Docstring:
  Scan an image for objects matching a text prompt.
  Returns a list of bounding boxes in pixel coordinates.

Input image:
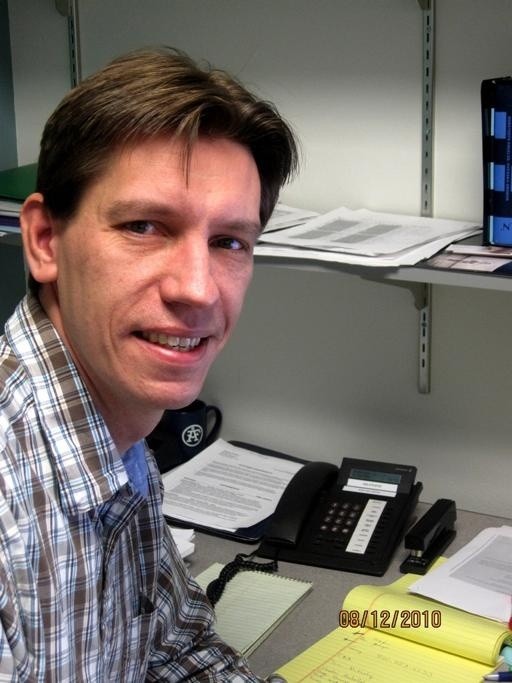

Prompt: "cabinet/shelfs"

[0,1,511,394]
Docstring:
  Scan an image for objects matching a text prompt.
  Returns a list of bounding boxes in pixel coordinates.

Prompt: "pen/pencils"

[482,671,512,683]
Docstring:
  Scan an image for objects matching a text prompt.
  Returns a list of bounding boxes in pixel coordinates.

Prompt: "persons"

[1,47,298,682]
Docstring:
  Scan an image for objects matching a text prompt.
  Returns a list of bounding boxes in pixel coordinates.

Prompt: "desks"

[162,438,512,683]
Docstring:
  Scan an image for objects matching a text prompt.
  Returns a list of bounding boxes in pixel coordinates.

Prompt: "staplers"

[399,498,457,575]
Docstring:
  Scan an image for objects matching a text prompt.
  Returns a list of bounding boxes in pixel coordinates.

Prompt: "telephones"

[205,456,424,607]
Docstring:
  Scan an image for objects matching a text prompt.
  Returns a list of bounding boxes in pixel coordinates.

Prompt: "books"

[485,95,512,246]
[2,163,40,219]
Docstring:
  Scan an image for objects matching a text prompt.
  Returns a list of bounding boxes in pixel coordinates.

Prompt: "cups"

[172,400,222,461]
